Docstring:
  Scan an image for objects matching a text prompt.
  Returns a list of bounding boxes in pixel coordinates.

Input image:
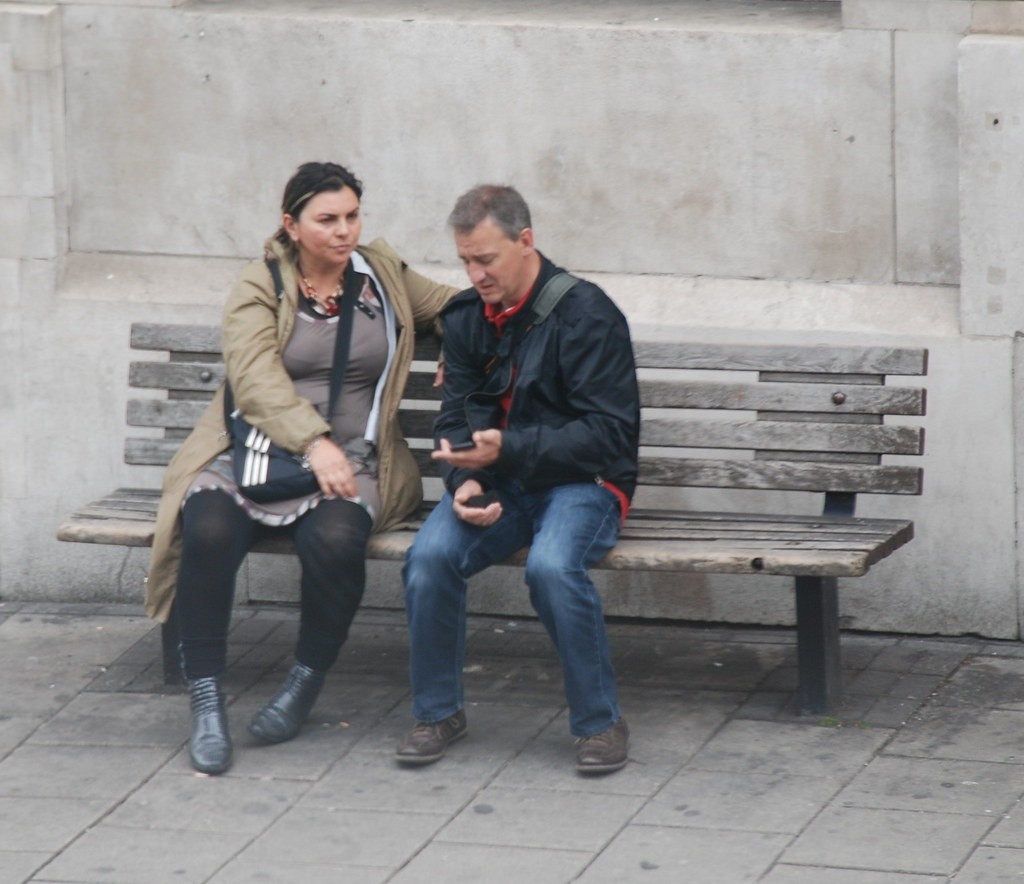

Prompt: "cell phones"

[449,442,474,452]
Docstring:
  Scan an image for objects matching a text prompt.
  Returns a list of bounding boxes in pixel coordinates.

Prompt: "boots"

[247,663,326,743]
[187,674,234,772]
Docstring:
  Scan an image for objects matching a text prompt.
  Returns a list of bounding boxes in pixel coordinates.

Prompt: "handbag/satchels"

[228,403,320,504]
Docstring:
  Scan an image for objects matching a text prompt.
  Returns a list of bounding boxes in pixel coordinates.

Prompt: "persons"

[395,187,641,773]
[144,162,464,774]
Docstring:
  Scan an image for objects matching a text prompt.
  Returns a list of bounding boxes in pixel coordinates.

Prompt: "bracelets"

[301,436,323,468]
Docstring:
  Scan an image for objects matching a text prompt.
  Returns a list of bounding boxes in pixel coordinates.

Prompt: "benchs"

[62,319,928,707]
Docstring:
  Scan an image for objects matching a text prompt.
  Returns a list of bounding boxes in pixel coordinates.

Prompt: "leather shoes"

[574,717,630,771]
[395,709,469,761]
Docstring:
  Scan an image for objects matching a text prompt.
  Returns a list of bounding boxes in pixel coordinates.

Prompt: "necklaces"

[296,262,346,309]
